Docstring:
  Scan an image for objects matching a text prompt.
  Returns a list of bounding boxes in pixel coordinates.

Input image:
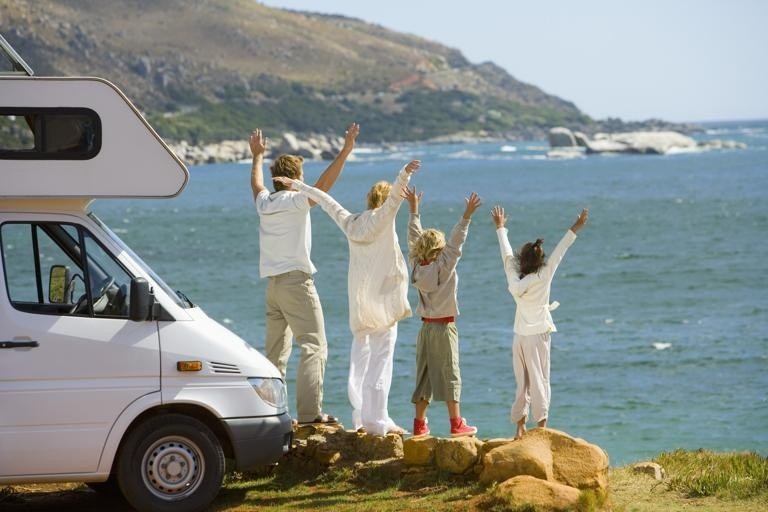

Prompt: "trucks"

[0,33,295,509]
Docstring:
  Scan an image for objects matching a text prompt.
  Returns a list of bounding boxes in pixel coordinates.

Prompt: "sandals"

[298,414,339,425]
[387,424,411,436]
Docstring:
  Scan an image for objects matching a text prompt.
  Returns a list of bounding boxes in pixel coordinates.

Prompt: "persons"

[398,183,484,437]
[269,158,421,436]
[246,121,360,424]
[489,205,590,440]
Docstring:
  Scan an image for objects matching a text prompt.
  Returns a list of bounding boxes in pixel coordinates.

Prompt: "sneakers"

[413,417,431,437]
[449,416,477,437]
